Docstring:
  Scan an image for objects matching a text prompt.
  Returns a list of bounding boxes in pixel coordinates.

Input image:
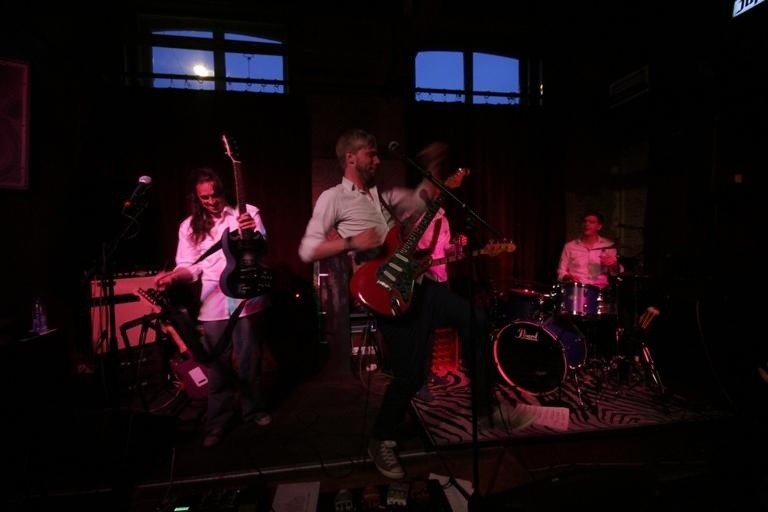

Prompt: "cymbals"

[589,241,637,251]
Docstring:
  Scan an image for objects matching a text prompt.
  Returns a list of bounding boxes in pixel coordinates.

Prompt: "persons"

[148,164,274,449]
[298,127,492,477]
[552,207,621,394]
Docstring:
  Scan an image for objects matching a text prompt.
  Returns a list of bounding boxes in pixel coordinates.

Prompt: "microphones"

[122,174,153,208]
[614,222,637,232]
[389,139,432,178]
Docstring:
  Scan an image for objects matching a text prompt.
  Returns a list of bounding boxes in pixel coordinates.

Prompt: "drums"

[558,282,598,322]
[495,288,557,326]
[597,288,618,317]
[493,318,587,396]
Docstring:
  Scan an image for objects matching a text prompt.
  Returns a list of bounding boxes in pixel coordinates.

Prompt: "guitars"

[220,134,274,298]
[350,169,471,319]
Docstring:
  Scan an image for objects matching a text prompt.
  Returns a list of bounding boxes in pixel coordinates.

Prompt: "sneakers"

[203,430,218,447]
[254,413,271,426]
[367,441,405,480]
